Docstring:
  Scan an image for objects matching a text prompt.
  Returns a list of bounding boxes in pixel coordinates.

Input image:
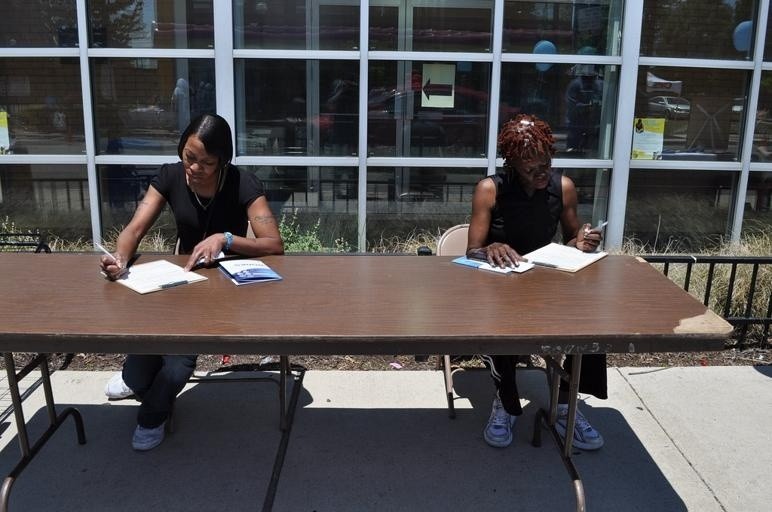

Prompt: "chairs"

[165,221,291,431]
[436,224,553,418]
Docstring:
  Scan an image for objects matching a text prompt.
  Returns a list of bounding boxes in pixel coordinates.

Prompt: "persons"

[467,114,607,450]
[566,72,605,149]
[99,113,284,451]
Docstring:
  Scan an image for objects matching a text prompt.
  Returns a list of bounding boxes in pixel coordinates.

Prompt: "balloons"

[532,38,558,73]
[732,20,753,51]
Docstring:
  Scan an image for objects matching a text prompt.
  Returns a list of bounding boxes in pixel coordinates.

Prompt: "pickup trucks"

[2,101,303,216]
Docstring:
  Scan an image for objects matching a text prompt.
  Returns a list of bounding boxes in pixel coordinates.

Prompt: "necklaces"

[189,168,222,210]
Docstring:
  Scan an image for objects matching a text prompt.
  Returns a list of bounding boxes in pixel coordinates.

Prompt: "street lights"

[530,37,560,97]
[730,19,754,106]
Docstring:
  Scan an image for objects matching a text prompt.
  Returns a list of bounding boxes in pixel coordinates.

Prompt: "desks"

[0,250,733,512]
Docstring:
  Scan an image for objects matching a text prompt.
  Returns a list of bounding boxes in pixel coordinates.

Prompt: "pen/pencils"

[584,221,609,235]
[95,242,130,274]
[532,262,556,268]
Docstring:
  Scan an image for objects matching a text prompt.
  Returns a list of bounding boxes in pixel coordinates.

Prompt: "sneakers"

[483,391,519,451]
[129,420,169,452]
[546,402,607,455]
[103,371,136,400]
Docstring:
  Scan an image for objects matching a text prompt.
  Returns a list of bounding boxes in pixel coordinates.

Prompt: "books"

[450,251,537,275]
[214,257,282,286]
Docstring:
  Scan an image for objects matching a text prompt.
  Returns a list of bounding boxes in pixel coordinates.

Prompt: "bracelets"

[224,232,233,252]
